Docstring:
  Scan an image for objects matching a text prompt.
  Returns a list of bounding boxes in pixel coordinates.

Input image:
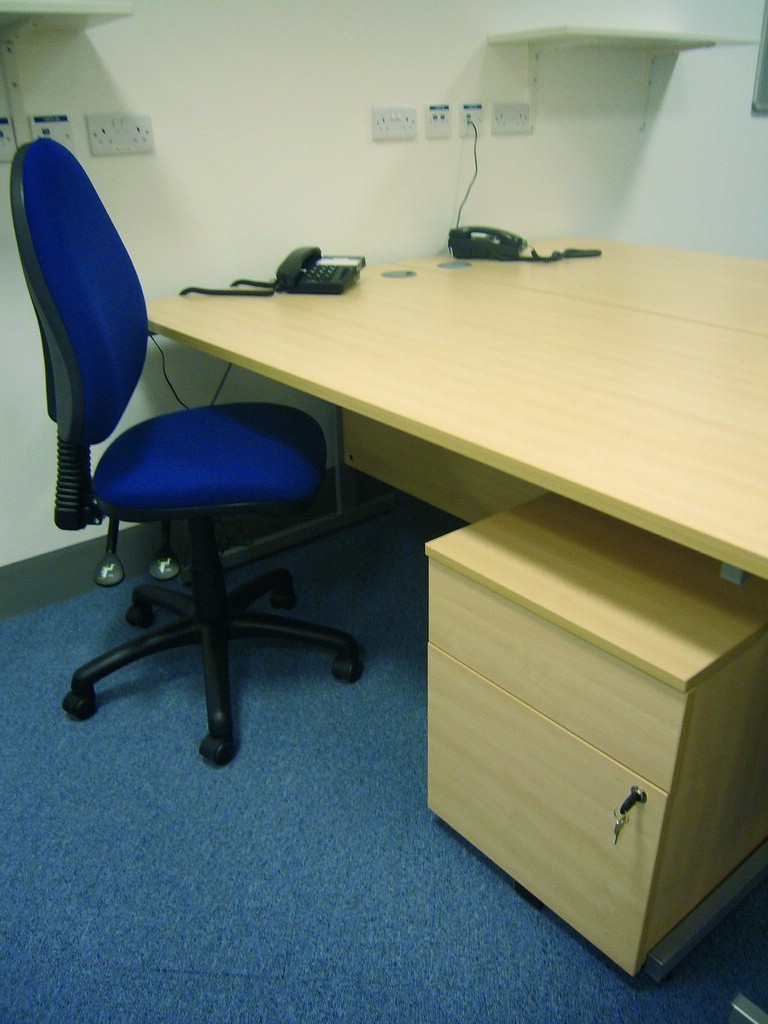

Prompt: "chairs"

[10,138,367,768]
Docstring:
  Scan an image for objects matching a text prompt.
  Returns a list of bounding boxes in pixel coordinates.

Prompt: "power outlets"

[458,102,483,138]
[85,113,155,158]
[489,102,530,135]
[371,106,417,141]
[35,114,73,149]
[426,103,451,138]
[0,116,15,162]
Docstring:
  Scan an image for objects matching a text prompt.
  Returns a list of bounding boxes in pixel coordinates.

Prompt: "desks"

[142,247,768,581]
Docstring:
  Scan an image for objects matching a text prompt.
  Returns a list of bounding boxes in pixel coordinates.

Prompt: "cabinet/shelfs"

[422,493,768,978]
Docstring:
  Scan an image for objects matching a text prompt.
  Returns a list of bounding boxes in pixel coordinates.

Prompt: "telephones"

[275,246,368,298]
[448,224,523,262]
